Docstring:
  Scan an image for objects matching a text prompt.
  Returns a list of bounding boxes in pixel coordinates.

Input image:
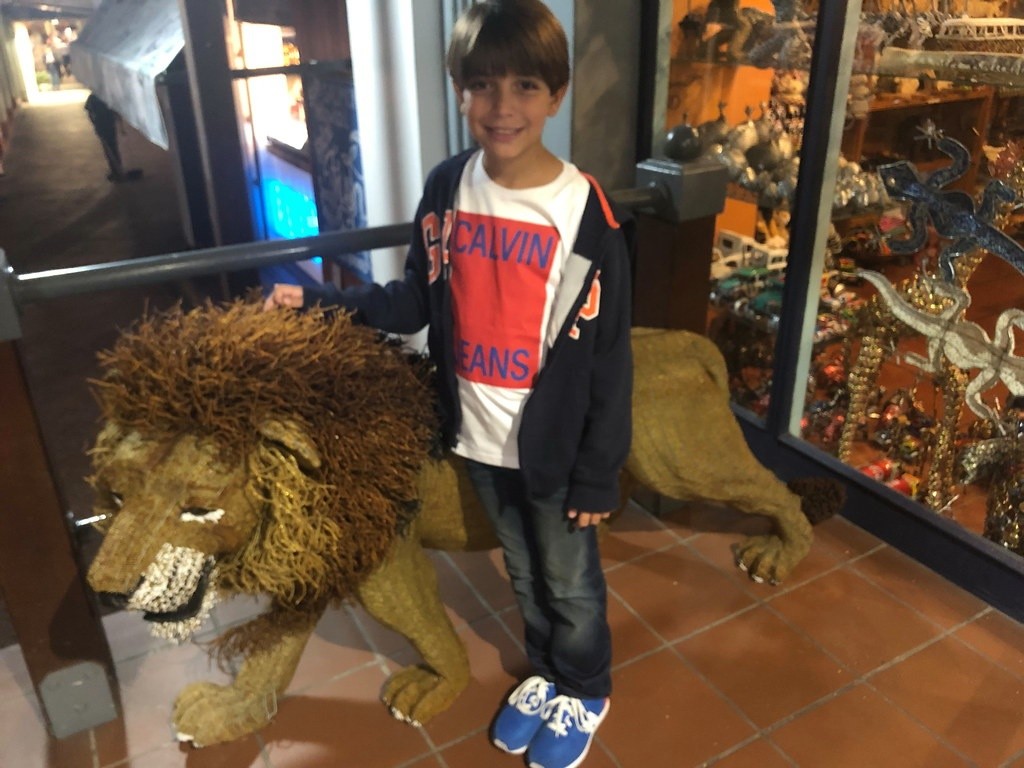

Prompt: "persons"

[42,24,78,91]
[85,93,125,174]
[261,1,634,768]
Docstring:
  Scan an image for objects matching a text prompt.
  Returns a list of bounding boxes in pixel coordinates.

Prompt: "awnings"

[69,0,186,151]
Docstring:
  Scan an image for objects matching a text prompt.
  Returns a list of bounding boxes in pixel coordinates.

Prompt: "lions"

[87,288,845,750]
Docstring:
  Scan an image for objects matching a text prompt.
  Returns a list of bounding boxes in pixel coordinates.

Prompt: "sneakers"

[494,673,560,755]
[527,691,610,768]
